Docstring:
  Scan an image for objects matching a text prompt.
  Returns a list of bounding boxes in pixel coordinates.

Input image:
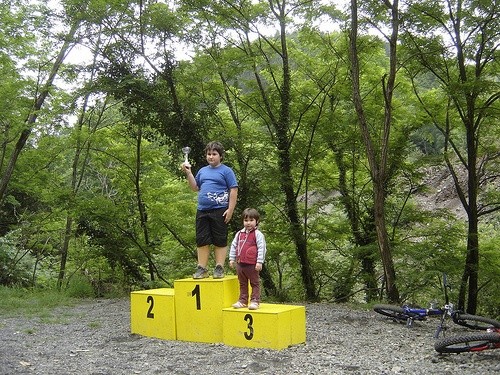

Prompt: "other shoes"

[193,265,209,278]
[213,263,224,278]
[248,302,260,310]
[232,302,248,309]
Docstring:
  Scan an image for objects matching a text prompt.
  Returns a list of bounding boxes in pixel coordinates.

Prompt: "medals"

[236,256,240,264]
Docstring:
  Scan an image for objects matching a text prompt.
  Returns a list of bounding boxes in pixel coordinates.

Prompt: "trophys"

[181,147,192,169]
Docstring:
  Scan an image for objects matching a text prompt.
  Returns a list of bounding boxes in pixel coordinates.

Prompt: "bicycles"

[434,334,500,354]
[372,273,500,338]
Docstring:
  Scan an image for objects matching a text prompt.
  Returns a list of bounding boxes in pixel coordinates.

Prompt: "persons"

[228,208,267,310]
[181,141,239,279]
